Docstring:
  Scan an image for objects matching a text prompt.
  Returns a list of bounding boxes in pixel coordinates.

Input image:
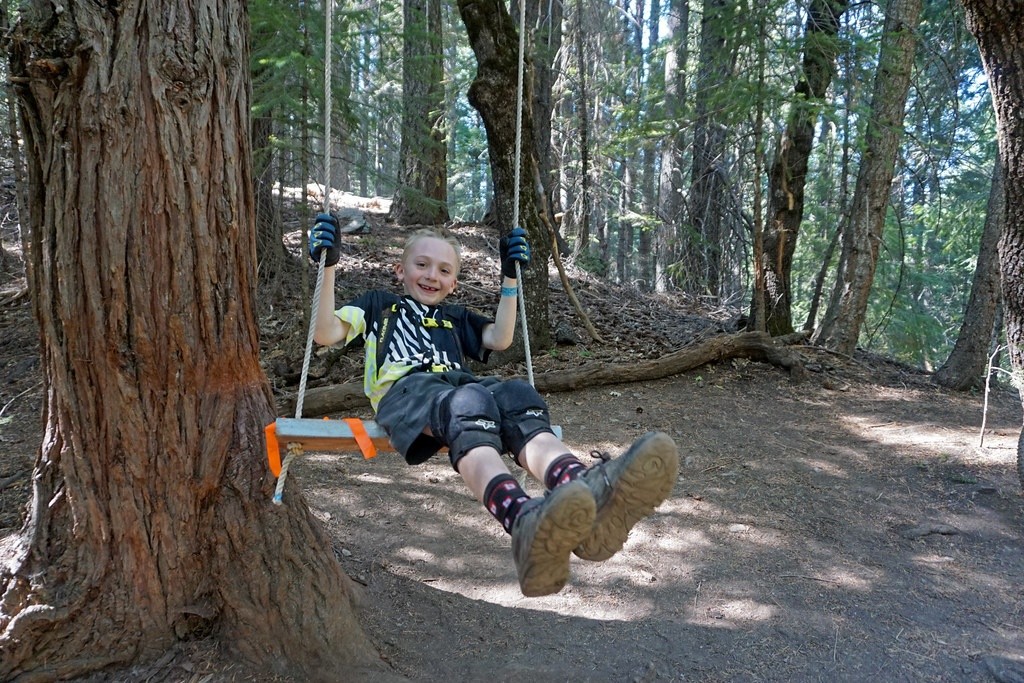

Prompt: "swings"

[264,1,565,456]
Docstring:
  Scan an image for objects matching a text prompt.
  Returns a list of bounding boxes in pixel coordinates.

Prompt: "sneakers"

[512,480,597,597]
[572,430,678,562]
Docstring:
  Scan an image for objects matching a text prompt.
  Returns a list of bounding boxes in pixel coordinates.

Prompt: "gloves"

[309,210,341,267]
[499,227,531,279]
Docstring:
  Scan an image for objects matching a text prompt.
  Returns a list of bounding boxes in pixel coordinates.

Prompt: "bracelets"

[501,283,518,297]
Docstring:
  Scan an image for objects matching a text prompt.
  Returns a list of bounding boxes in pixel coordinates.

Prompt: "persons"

[310,212,678,597]
